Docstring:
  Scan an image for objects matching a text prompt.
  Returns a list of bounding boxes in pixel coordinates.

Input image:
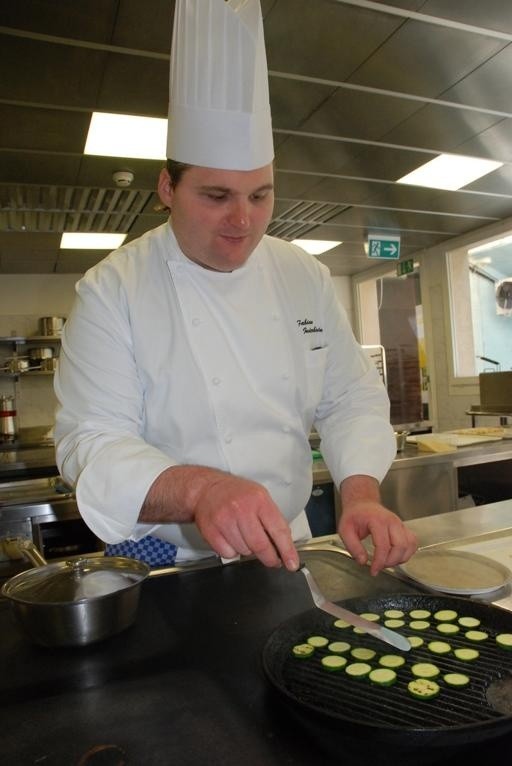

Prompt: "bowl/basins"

[37,316,66,336]
[394,428,410,453]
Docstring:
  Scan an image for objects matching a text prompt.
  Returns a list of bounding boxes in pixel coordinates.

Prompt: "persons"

[52,154,418,580]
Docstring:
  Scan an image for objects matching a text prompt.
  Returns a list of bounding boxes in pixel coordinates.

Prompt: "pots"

[18,356,59,371]
[260,591,512,745]
[4,346,54,361]
[1,537,154,653]
[0,352,31,374]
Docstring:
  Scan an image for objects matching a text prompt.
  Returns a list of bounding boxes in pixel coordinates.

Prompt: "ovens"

[28,508,108,567]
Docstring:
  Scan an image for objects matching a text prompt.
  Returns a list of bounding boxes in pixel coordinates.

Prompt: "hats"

[166,0,275,171]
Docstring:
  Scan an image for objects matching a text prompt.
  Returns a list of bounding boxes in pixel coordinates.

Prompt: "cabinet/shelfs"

[0,335,62,376]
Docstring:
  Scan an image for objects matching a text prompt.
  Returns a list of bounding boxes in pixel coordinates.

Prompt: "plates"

[396,548,511,596]
